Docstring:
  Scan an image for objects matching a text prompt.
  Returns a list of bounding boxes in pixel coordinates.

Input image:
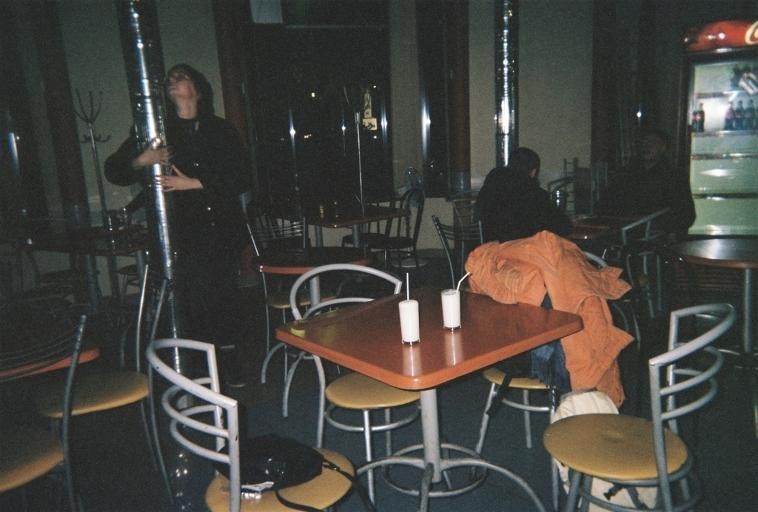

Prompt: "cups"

[396,299,421,346]
[445,333,466,367]
[440,289,462,330]
[400,347,422,379]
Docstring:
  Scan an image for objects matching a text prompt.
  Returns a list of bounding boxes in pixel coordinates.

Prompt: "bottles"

[105,204,130,232]
[722,98,757,130]
[692,104,707,133]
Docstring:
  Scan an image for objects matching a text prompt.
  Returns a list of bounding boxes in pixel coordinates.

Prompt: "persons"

[473,146,577,244]
[592,124,695,282]
[104,64,251,387]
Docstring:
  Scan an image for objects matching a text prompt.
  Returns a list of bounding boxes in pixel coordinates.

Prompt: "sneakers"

[216,345,248,389]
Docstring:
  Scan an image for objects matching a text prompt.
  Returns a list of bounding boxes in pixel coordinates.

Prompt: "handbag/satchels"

[210,430,325,496]
